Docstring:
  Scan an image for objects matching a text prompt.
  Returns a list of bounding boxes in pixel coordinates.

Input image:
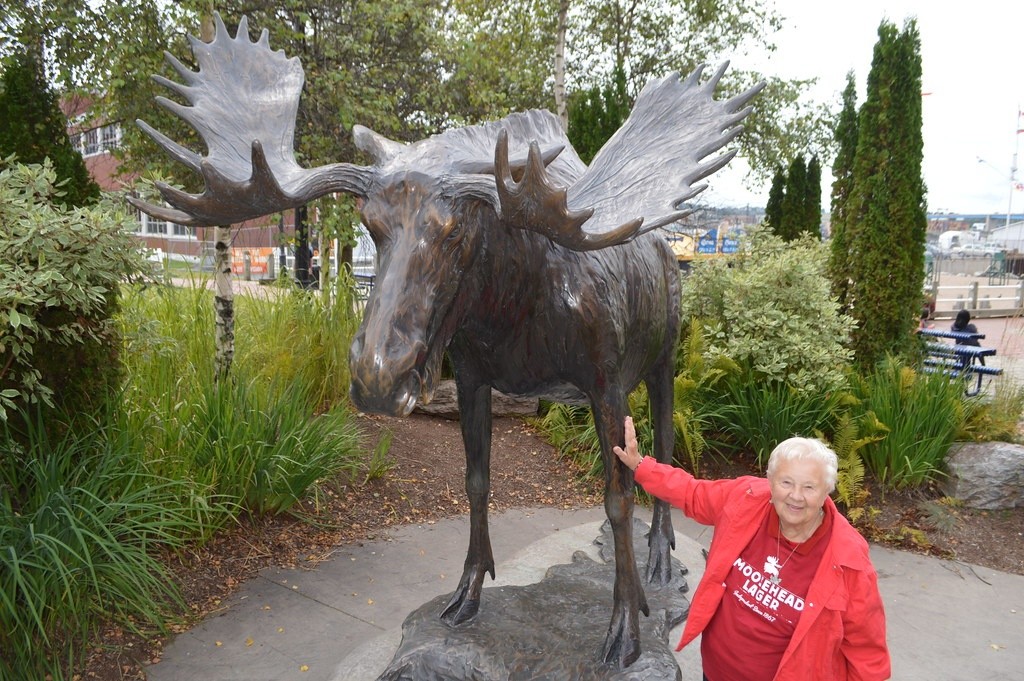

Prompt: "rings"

[635,435,637,438]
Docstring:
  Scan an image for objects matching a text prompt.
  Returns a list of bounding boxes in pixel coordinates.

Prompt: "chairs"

[330,275,374,297]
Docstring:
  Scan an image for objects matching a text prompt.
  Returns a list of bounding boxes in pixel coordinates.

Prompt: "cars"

[922,227,1024,259]
[266,254,376,281]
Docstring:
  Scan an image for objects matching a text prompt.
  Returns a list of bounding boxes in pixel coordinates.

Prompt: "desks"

[354,272,377,299]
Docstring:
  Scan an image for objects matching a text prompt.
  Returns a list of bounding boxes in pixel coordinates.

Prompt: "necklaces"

[769,506,823,588]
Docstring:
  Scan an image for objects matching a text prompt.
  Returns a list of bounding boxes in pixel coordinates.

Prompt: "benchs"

[917,328,1004,396]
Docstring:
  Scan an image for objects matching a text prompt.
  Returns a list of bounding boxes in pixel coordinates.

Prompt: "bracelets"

[634,457,644,470]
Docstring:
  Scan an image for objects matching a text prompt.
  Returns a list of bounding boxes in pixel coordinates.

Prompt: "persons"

[951,310,979,379]
[916,307,940,343]
[613,416,891,681]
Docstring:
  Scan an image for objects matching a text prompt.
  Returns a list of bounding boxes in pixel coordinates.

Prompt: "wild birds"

[123,8,770,667]
